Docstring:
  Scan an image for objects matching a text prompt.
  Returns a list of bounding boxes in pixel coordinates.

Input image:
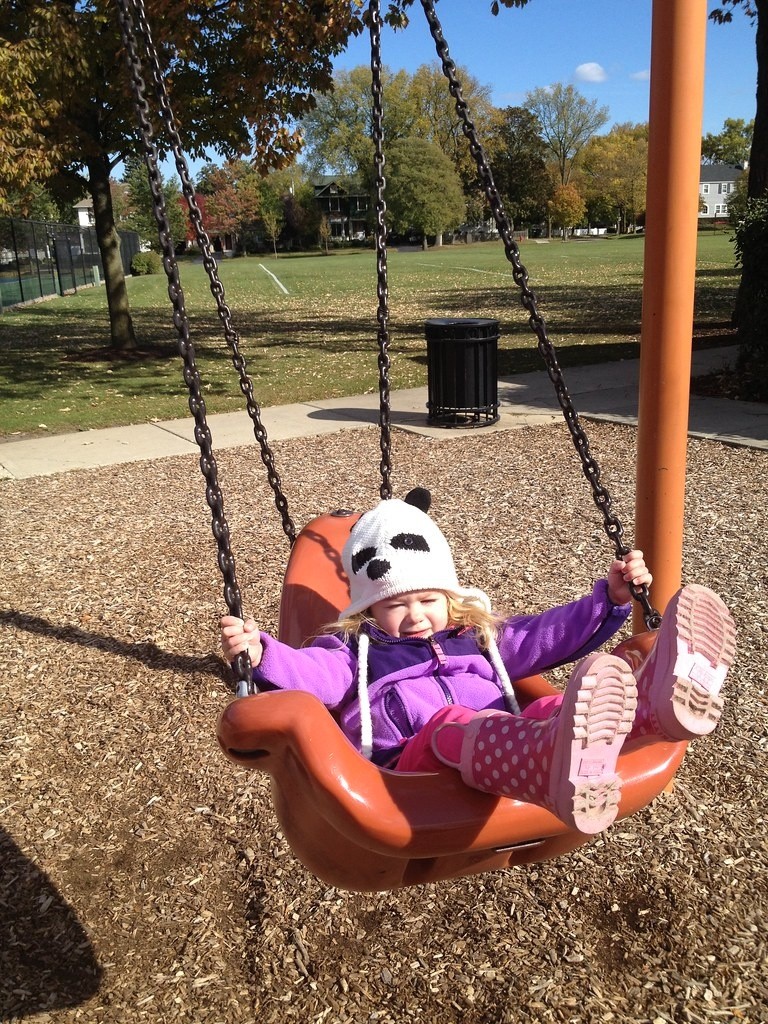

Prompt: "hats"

[338,487,491,624]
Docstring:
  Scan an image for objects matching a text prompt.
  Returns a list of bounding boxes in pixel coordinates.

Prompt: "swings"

[116,0,691,891]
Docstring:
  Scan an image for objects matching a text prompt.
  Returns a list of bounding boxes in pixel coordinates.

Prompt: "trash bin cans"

[424,317,502,429]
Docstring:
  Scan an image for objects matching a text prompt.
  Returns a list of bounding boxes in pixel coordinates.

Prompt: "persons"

[222,487,737,834]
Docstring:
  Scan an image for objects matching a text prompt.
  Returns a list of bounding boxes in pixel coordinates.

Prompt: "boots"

[548,582,737,743]
[429,652,639,835]
